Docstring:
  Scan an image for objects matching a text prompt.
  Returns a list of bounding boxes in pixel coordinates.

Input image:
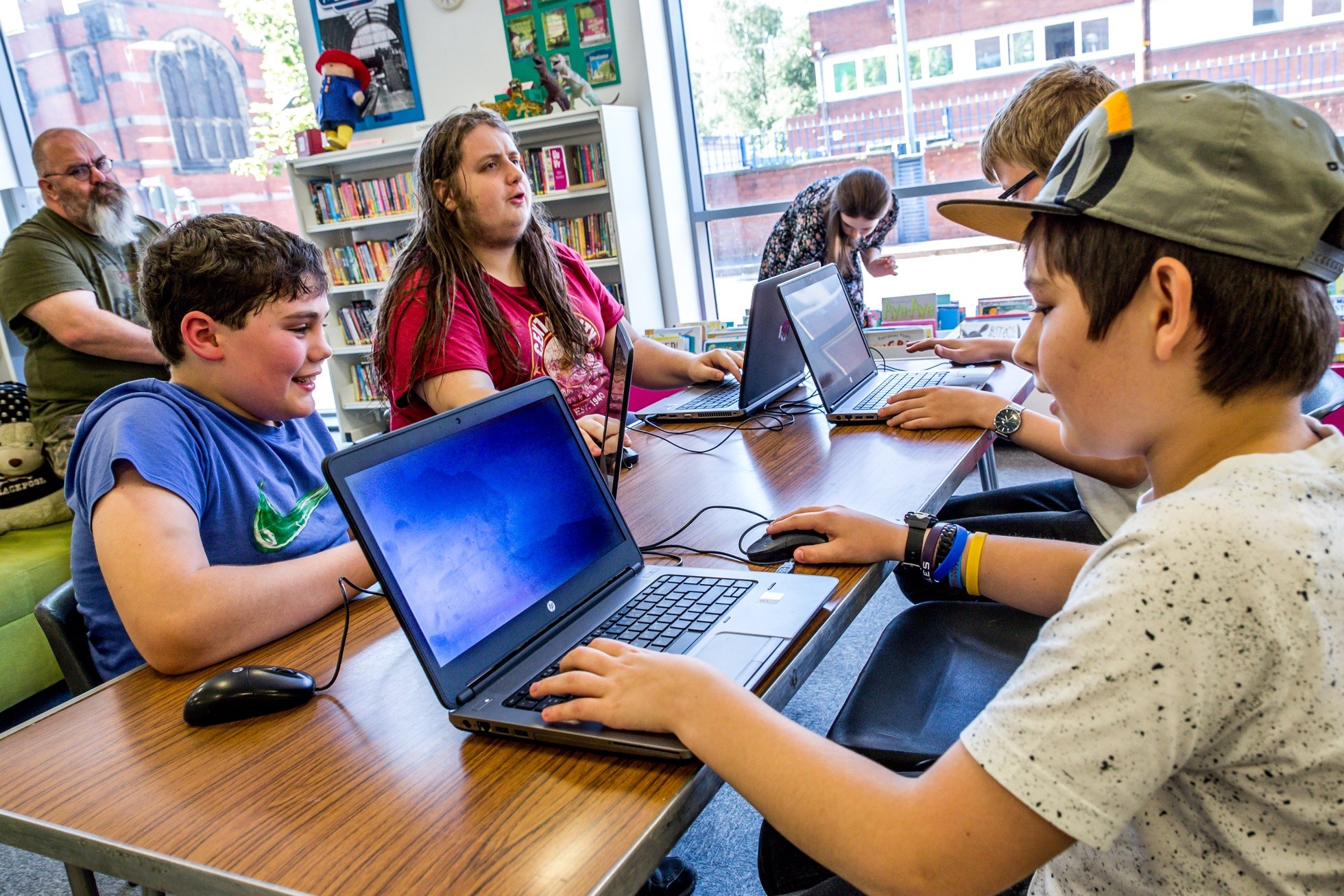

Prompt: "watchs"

[901,510,940,575]
[992,402,1027,442]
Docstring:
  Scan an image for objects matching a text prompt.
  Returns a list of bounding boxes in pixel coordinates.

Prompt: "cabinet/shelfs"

[286,102,665,444]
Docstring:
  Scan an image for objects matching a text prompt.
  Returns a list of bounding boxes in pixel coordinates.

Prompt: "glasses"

[43,157,113,181]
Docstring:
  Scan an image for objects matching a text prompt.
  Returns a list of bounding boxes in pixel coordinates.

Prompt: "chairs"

[828,601,1043,767]
[34,579,102,696]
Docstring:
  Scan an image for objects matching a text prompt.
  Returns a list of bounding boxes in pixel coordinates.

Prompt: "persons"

[0,125,170,476]
[877,61,1154,543]
[67,215,376,676]
[528,79,1344,895]
[757,167,900,328]
[371,107,745,457]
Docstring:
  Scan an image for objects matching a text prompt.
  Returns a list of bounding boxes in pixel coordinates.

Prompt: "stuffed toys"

[0,380,74,536]
[315,49,371,150]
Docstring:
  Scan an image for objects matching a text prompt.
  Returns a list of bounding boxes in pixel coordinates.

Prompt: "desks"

[0,361,1034,896]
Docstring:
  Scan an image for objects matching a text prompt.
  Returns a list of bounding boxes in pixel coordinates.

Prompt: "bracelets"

[921,522,987,597]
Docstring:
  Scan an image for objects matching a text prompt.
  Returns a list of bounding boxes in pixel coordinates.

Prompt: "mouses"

[950,352,1002,365]
[182,664,315,727]
[746,529,829,565]
[591,440,639,470]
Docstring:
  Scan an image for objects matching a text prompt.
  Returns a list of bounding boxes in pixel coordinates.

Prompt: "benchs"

[0,521,78,713]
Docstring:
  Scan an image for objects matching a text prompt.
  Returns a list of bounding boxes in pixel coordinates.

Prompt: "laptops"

[633,261,822,424]
[776,262,995,424]
[319,375,840,761]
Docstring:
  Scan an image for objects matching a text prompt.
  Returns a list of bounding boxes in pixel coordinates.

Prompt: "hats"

[936,79,1344,283]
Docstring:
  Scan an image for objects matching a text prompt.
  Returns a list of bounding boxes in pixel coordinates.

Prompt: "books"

[641,313,749,356]
[862,290,1036,349]
[307,143,623,403]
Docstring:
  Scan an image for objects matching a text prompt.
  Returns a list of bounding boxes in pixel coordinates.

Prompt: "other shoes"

[635,856,697,896]
